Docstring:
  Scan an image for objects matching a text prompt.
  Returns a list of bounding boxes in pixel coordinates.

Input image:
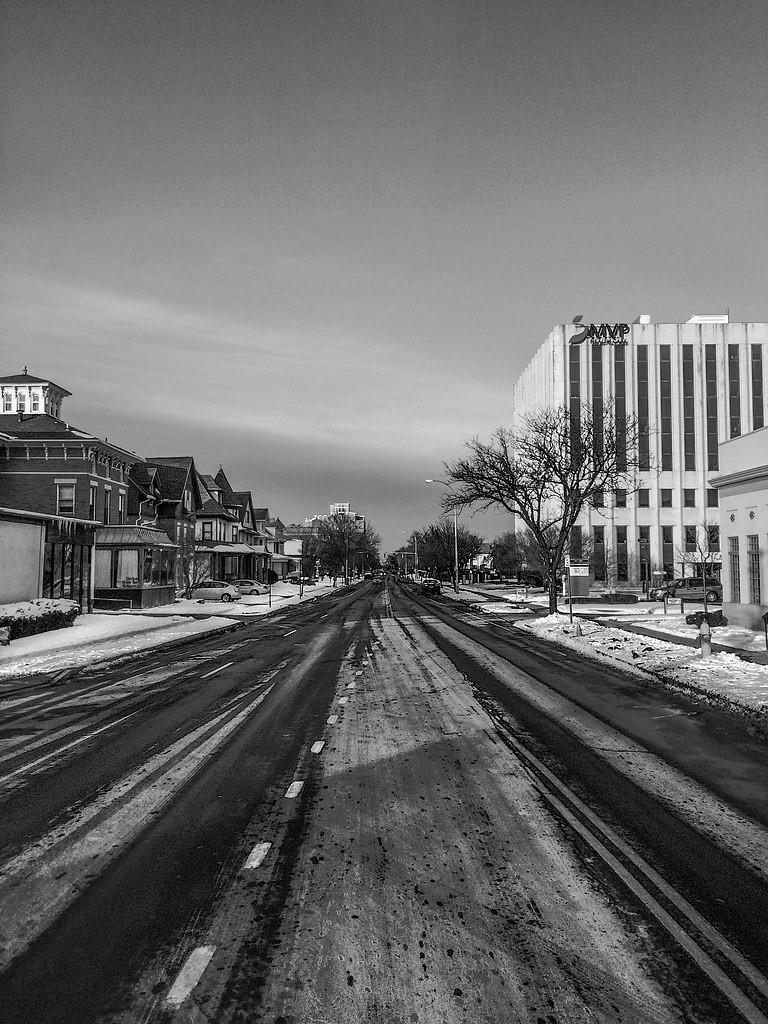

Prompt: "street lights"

[424,479,460,593]
[401,532,417,577]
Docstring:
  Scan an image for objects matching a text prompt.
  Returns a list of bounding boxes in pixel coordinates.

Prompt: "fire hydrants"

[697,618,712,657]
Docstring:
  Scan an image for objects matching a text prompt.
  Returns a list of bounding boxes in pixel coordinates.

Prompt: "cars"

[379,572,387,577]
[372,578,382,585]
[284,576,298,584]
[420,579,441,594]
[651,576,723,602]
[364,573,372,580]
[176,579,241,602]
[229,579,270,596]
